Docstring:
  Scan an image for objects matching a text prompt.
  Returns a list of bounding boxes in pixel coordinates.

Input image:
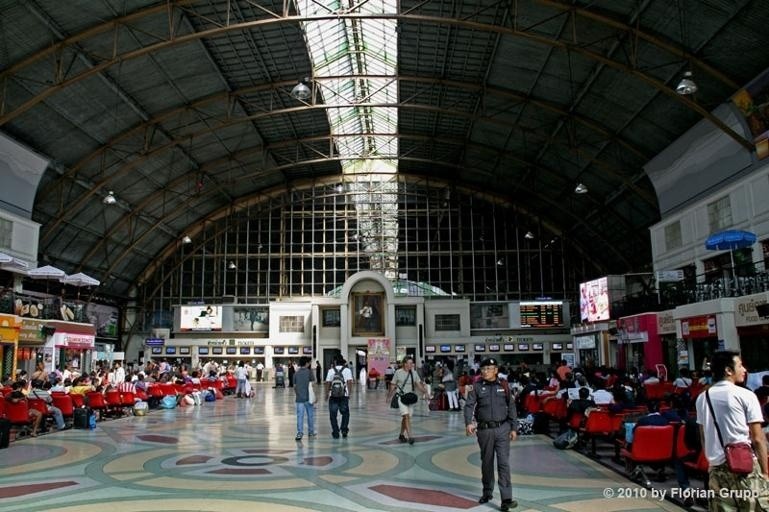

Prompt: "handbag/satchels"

[123,387,225,416]
[517,415,534,436]
[400,392,419,406]
[389,394,399,409]
[723,443,755,475]
[307,381,317,405]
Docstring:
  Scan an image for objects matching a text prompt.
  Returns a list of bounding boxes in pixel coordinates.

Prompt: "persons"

[596,284,608,319]
[580,286,590,323]
[328,350,768,482]
[293,355,318,440]
[313,360,322,386]
[695,350,769,512]
[0,354,295,438]
[359,300,373,332]
[324,354,354,439]
[182,304,269,333]
[463,359,519,512]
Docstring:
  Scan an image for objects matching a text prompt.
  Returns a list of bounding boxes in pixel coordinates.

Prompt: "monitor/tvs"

[474,344,543,352]
[166,347,176,353]
[199,347,264,354]
[180,347,190,354]
[303,347,312,354]
[274,347,284,354]
[552,342,573,350]
[152,347,162,354]
[288,347,299,354]
[454,344,464,352]
[440,345,450,352]
[425,345,436,353]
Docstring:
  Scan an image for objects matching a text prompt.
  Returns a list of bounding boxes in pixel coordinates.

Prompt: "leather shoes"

[500,497,518,512]
[479,494,495,504]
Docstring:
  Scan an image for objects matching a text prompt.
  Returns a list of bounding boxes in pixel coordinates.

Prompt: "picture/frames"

[152,346,312,354]
[425,342,573,352]
[350,291,385,336]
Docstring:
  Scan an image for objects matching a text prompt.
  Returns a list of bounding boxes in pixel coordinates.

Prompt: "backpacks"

[330,365,348,399]
[552,428,579,450]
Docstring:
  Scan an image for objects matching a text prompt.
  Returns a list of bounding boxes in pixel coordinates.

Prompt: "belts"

[481,415,511,429]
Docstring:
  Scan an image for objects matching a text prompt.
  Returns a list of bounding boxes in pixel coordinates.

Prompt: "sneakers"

[332,430,349,439]
[408,437,414,444]
[399,432,406,443]
[57,424,72,430]
[308,432,318,437]
[295,433,302,440]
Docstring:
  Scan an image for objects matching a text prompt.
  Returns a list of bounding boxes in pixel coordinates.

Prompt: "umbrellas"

[0,252,28,273]
[705,229,757,276]
[28,264,67,298]
[59,272,100,301]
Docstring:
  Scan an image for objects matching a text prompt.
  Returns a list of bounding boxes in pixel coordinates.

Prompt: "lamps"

[182,235,192,244]
[574,181,588,194]
[524,230,534,239]
[228,261,236,269]
[497,257,505,265]
[102,190,117,205]
[675,67,698,94]
[290,76,311,100]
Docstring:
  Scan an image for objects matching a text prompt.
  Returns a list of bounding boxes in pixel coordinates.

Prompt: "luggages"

[72,407,88,430]
[533,412,550,435]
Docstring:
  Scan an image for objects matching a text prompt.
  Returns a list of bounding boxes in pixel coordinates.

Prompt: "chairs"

[525,381,769,512]
[0,374,237,438]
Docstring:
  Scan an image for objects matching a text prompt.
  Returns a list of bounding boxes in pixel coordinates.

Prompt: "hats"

[479,358,497,367]
